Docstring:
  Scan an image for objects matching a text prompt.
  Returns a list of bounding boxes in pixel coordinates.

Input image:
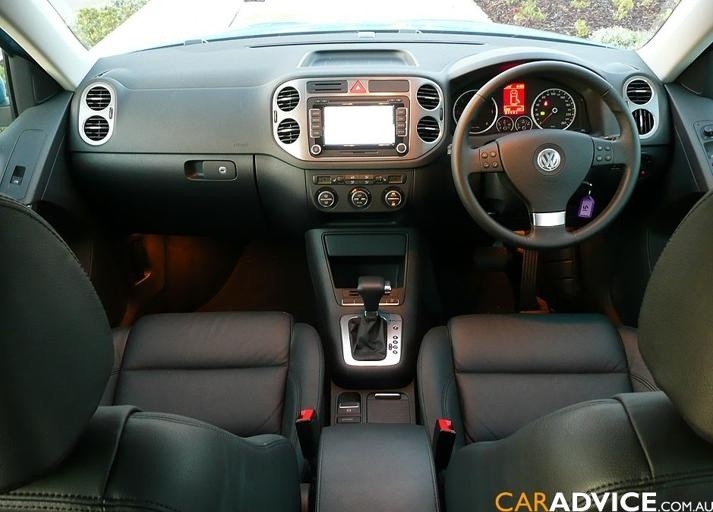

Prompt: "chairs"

[0,192,713,512]
